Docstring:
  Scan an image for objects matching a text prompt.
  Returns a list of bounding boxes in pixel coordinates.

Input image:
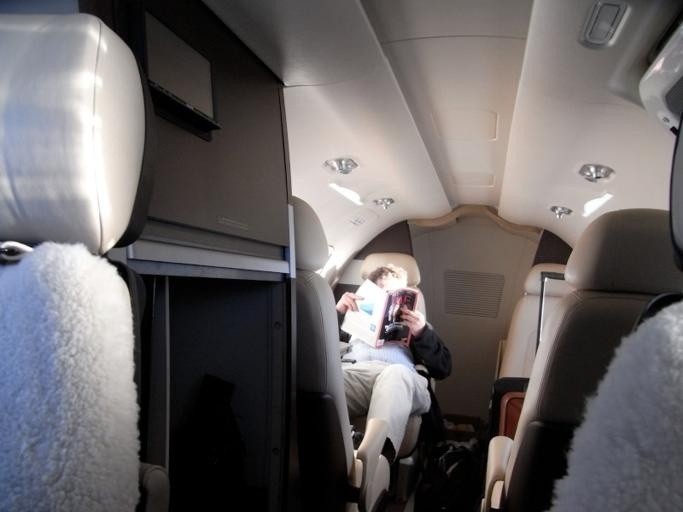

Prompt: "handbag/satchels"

[413,443,477,511]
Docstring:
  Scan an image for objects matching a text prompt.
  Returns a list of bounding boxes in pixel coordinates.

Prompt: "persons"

[336,263,452,466]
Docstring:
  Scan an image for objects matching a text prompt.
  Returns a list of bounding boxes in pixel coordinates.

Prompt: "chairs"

[0,12,146,512]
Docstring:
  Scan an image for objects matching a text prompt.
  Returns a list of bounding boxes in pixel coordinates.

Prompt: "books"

[339,278,419,350]
